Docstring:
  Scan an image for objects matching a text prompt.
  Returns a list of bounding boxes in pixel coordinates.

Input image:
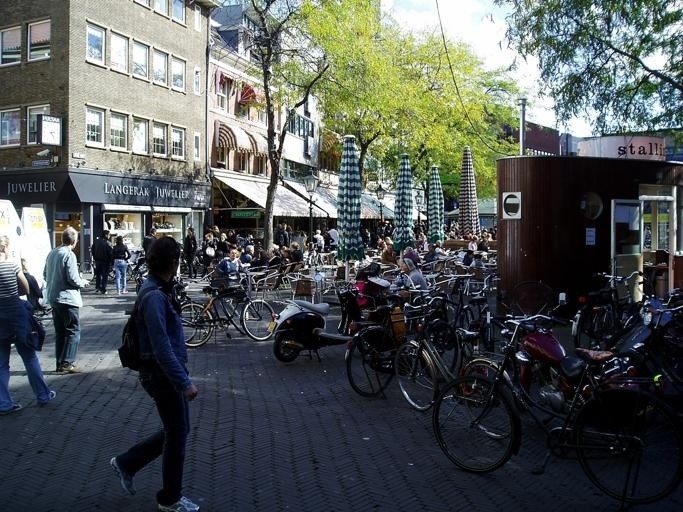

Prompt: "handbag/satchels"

[206,246,215,256]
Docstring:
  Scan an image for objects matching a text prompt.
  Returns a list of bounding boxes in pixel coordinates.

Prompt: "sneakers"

[56,363,83,372]
[159,497,200,512]
[111,458,136,497]
[50,391,56,400]
[0,405,22,415]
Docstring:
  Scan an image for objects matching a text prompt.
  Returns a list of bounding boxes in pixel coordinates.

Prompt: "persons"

[19,258,43,315]
[251,222,339,293]
[90,216,254,293]
[0,232,56,417]
[41,225,90,374]
[110,232,202,511]
[366,220,494,290]
[643,224,652,250]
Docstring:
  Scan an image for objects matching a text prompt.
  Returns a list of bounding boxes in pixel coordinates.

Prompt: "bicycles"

[430,314,683,512]
[79,247,149,296]
[172,243,683,441]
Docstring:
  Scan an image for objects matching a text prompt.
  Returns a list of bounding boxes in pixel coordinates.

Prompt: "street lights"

[414,190,424,227]
[375,184,387,241]
[302,168,320,251]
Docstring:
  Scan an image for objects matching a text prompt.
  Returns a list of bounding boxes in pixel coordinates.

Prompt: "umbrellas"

[458,144,484,240]
[337,133,365,287]
[393,153,417,264]
[426,163,445,251]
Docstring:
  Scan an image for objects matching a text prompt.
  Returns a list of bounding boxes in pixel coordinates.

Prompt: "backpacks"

[118,287,169,370]
